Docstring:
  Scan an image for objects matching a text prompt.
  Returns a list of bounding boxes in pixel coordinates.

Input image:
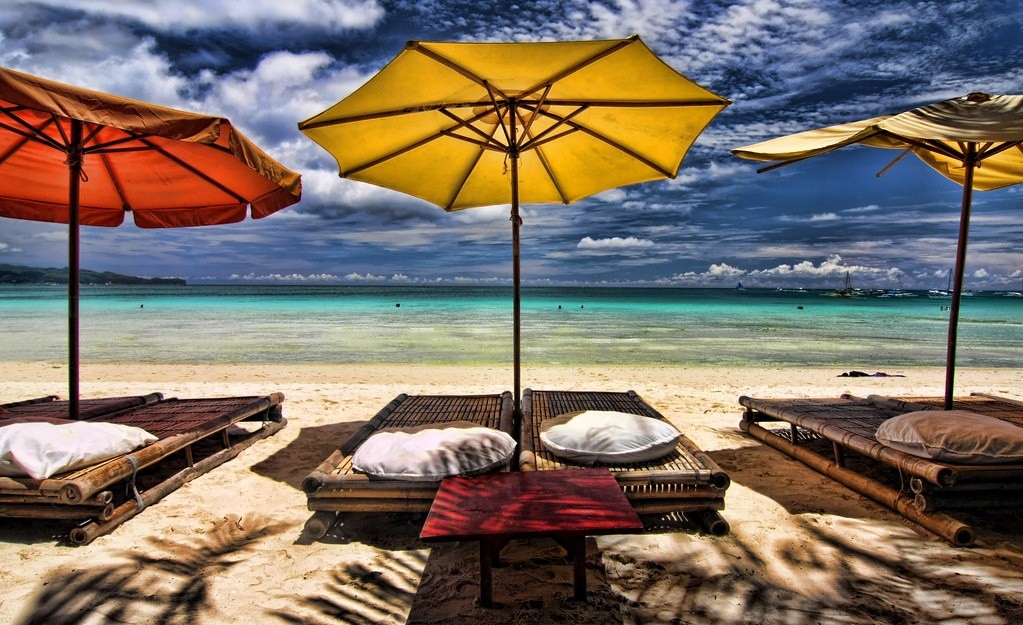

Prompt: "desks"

[420,467,643,610]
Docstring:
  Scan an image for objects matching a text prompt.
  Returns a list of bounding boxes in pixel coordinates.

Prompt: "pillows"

[350,420,517,482]
[0,417,159,482]
[540,410,685,467]
[874,409,1023,466]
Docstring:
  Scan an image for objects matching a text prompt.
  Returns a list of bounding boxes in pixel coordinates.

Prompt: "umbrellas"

[298,35,732,426]
[734,92,1023,412]
[0,66,302,421]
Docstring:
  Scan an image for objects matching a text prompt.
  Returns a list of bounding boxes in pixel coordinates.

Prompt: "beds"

[0,391,288,543]
[738,391,1023,545]
[521,386,730,535]
[301,391,513,540]
